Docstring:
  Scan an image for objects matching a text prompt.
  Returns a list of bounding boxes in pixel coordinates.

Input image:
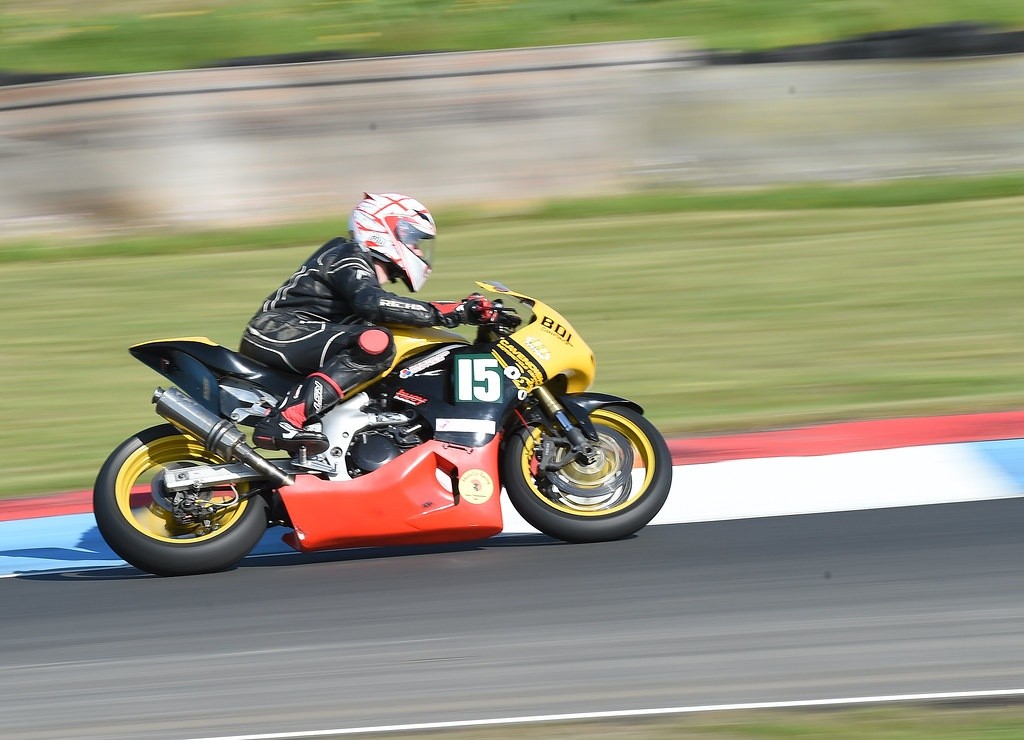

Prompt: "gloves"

[428,292,494,328]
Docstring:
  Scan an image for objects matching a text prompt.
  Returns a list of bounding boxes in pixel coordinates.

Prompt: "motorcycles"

[92,282,673,575]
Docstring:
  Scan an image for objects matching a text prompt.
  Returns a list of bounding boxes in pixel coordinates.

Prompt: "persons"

[239,190,498,455]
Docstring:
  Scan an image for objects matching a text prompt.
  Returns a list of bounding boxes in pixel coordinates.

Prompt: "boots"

[252,373,344,455]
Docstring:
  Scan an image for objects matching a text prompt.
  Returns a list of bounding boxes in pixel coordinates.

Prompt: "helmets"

[347,192,437,293]
